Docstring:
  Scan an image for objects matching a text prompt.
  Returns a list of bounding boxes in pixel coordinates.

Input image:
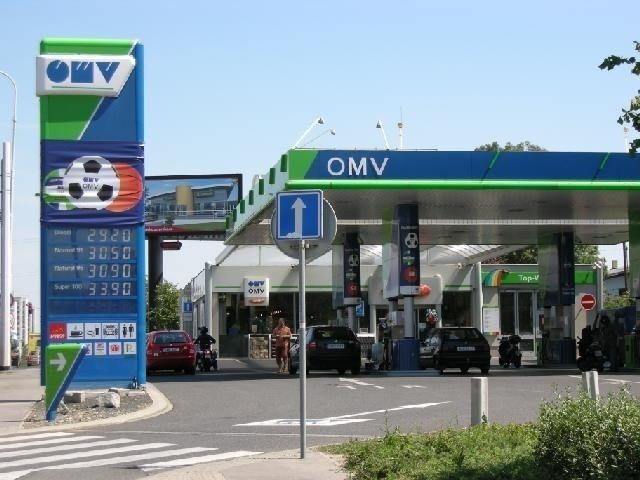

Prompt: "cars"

[421,326,494,379]
[27,350,42,366]
[145,330,197,377]
[285,323,363,375]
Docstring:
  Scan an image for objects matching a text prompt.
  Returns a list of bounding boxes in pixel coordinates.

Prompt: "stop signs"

[580,293,595,311]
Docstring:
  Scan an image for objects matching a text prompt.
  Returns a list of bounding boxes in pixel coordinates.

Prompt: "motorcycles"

[496,334,524,370]
[192,337,218,373]
[576,327,606,374]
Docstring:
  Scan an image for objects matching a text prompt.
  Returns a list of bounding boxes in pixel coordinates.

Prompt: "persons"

[195,327,214,347]
[272,318,292,371]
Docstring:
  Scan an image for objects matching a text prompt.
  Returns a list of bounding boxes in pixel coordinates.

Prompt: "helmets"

[200,326,209,334]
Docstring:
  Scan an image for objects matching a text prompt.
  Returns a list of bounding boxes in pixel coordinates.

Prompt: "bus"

[10,336,24,368]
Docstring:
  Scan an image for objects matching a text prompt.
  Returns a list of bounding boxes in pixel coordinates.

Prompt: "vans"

[593,307,628,373]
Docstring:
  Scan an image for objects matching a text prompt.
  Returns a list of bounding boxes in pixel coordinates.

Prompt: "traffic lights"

[27,302,33,316]
